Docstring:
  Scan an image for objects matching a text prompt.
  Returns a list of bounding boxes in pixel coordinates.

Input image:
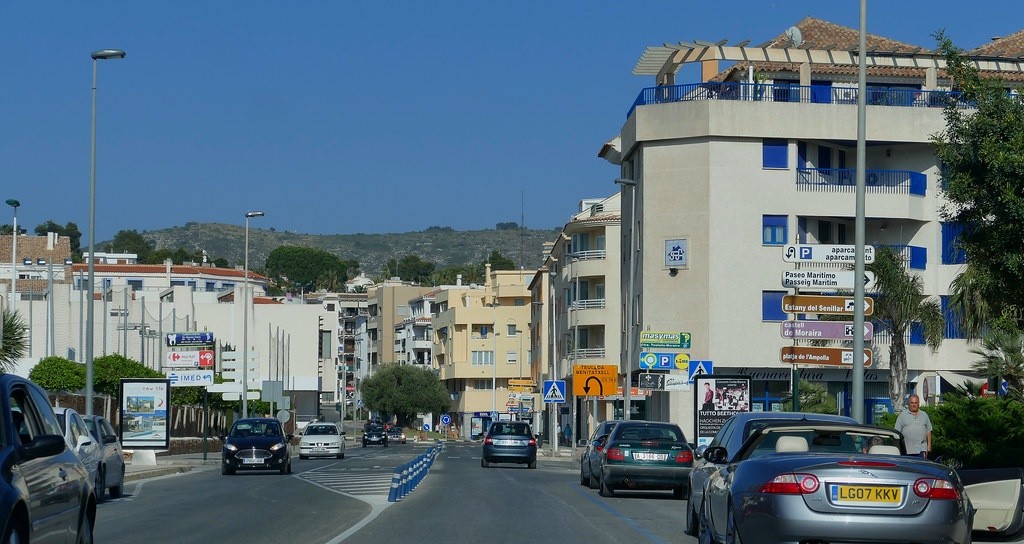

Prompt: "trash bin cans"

[420,430,428,441]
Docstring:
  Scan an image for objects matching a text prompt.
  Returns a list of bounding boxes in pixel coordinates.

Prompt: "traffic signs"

[780,346,873,368]
[782,294,874,316]
[779,319,873,342]
[781,269,874,290]
[505,379,533,414]
[783,243,875,265]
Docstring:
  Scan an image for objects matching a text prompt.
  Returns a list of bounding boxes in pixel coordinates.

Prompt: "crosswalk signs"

[543,380,566,404]
[688,360,714,383]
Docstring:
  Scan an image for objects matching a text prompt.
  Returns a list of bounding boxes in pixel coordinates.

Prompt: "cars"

[9,404,102,503]
[577,420,666,489]
[478,419,540,470]
[360,419,407,448]
[297,422,345,459]
[685,410,864,539]
[591,419,697,500]
[79,414,126,499]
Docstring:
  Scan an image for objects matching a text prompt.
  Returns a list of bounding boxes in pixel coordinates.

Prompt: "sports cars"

[700,419,1024,544]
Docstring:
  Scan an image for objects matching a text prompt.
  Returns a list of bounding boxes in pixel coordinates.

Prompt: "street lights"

[241,210,265,430]
[84,47,129,432]
[492,332,500,410]
[338,314,355,430]
[613,178,637,421]
[4,199,22,374]
[513,330,522,421]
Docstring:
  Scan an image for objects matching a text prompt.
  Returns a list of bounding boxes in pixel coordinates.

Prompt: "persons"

[557,423,562,445]
[894,394,933,460]
[704,383,713,404]
[862,436,884,454]
[435,423,455,435]
[563,424,572,446]
[716,386,748,409]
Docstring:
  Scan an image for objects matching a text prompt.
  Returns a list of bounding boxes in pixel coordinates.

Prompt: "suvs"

[219,417,294,475]
[0,373,98,544]
